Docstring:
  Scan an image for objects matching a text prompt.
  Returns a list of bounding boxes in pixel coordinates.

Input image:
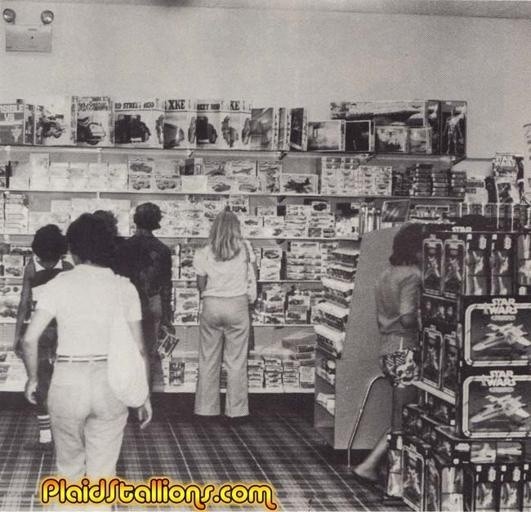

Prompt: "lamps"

[2,9,53,52]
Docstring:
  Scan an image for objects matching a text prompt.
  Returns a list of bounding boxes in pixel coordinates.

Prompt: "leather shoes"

[23,439,55,451]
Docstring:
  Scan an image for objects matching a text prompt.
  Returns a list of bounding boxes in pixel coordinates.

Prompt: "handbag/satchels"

[106,274,149,408]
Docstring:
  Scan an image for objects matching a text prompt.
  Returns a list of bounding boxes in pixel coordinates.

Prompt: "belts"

[54,354,107,365]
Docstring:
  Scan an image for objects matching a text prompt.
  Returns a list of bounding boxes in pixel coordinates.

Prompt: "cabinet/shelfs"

[314,222,409,467]
[0,145,471,395]
[403,229,531,512]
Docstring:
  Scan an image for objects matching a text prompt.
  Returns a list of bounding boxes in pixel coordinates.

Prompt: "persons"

[19,212,155,512]
[350,221,439,499]
[113,202,177,426]
[191,207,260,426]
[13,223,74,451]
[450,212,497,232]
[91,209,125,269]
